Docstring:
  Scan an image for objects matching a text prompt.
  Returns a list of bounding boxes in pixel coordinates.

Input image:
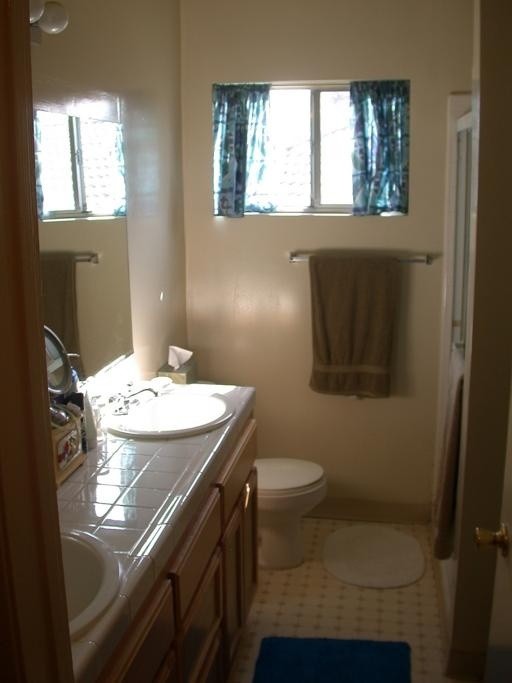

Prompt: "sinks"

[105,393,237,441]
[59,524,124,643]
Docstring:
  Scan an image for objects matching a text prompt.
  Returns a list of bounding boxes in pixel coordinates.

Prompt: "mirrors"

[36,106,136,385]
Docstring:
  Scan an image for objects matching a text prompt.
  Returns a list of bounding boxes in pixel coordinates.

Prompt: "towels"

[306,250,403,400]
[39,251,84,376]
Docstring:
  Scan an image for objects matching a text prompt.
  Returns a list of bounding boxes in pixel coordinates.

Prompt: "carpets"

[324,518,428,591]
[251,635,413,682]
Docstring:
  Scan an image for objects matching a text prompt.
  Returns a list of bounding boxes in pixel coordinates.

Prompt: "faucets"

[126,386,162,399]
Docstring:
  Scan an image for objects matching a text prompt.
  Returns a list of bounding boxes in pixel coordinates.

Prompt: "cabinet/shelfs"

[213,409,261,670]
[92,576,181,680]
[162,482,232,682]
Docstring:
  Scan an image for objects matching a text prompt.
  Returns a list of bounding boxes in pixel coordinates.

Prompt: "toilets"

[197,379,330,572]
